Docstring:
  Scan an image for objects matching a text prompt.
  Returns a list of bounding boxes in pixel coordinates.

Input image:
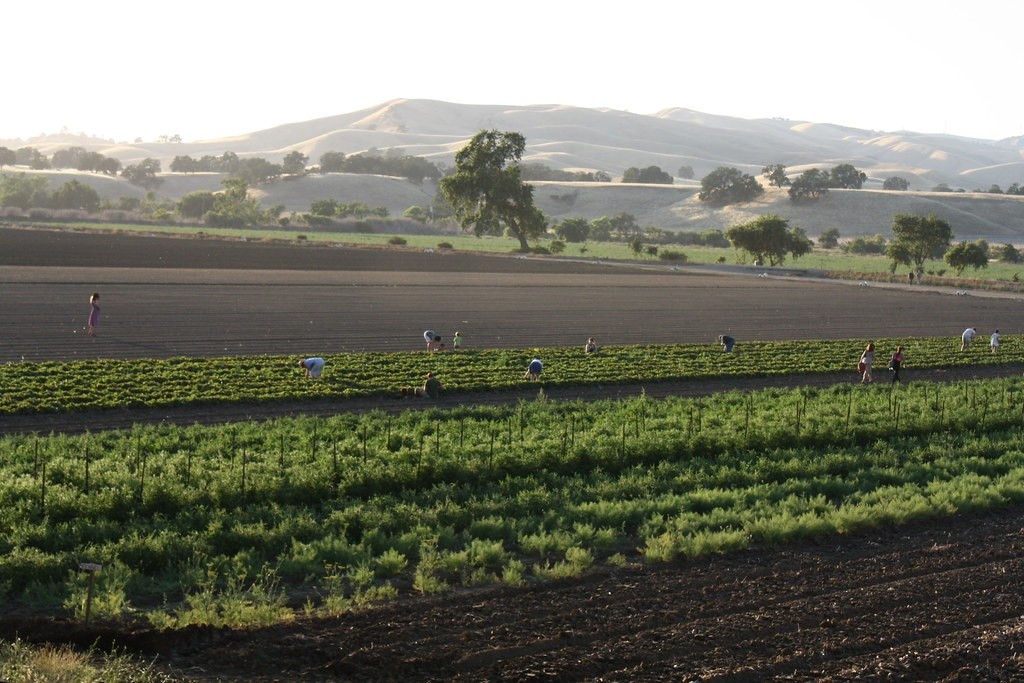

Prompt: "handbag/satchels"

[889,359,895,373]
[857,362,866,374]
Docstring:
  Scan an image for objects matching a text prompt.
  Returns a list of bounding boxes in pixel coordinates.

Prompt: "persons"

[917,269,923,286]
[719,334,736,353]
[890,345,906,384]
[438,343,446,351]
[523,357,545,381]
[585,337,598,352]
[415,372,445,399]
[299,357,325,381]
[87,292,101,337]
[960,327,977,353]
[908,269,915,286]
[454,331,462,350]
[424,330,442,351]
[989,330,1000,354]
[859,343,877,385]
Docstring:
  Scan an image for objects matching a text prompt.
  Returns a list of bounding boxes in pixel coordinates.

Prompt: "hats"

[428,373,433,377]
[299,360,304,366]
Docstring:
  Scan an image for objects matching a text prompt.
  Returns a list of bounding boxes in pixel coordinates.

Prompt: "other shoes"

[88,332,96,337]
